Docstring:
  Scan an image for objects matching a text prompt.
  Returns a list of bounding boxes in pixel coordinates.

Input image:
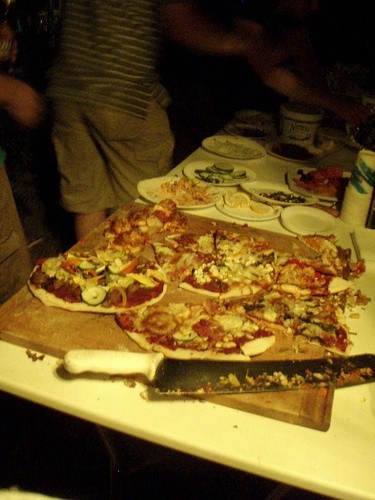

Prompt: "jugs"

[351,101,375,153]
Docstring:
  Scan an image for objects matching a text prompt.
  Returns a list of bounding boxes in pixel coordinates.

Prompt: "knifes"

[62,348,374,396]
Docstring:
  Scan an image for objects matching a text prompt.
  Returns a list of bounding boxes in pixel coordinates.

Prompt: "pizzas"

[27,198,371,360]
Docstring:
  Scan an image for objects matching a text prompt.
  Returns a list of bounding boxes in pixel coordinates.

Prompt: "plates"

[214,190,283,223]
[316,127,351,141]
[202,134,268,158]
[135,176,221,209]
[279,205,340,238]
[264,140,324,165]
[286,166,340,201]
[224,108,276,140]
[240,179,317,206]
[183,161,258,187]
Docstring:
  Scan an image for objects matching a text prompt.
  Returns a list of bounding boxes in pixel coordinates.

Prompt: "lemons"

[224,191,271,213]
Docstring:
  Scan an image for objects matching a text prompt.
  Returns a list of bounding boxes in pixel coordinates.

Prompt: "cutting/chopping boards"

[0,198,349,433]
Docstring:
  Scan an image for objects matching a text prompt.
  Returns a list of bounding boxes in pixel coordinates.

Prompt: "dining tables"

[0,124,375,500]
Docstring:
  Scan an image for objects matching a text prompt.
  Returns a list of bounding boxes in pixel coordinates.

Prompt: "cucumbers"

[195,162,247,184]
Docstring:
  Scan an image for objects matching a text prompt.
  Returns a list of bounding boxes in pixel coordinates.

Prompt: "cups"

[340,148,375,225]
[279,102,325,145]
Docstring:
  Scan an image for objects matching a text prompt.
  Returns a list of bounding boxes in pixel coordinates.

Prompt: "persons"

[44,0,265,241]
[0,74,46,307]
[234,21,369,125]
